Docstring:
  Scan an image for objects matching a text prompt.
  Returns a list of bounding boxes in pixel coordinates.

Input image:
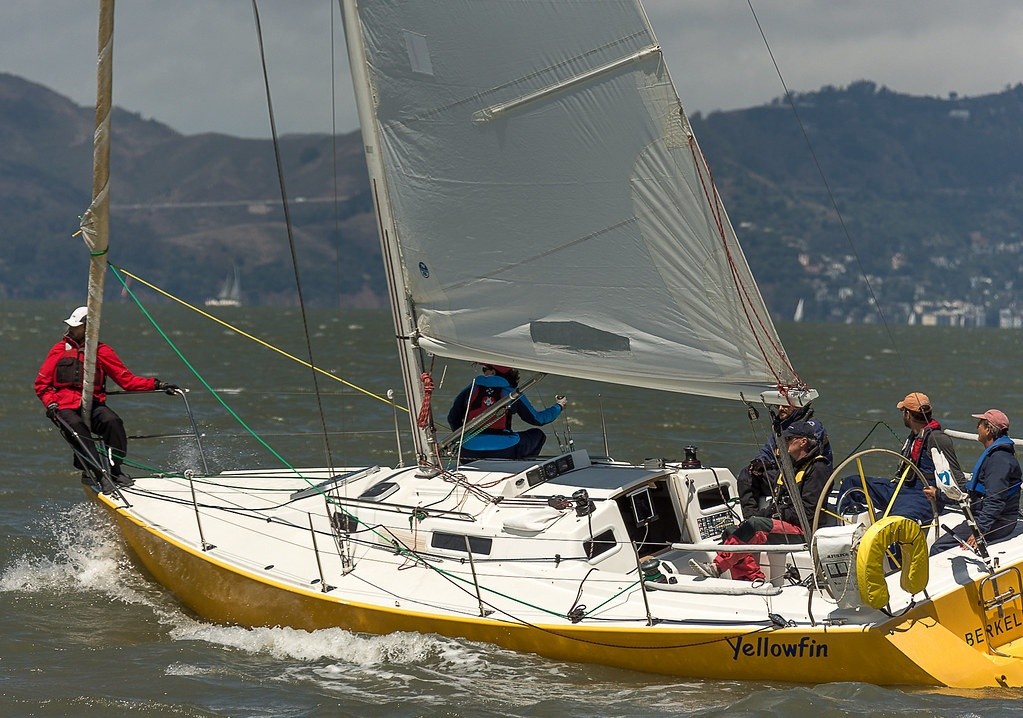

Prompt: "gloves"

[163,383,186,395]
[555,395,568,409]
[46,406,61,421]
[748,459,765,476]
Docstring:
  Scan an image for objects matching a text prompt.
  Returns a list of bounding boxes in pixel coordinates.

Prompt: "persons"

[688,422,833,584]
[445,364,568,462]
[922,408,1022,556]
[736,404,833,519]
[35,306,179,494]
[834,391,967,571]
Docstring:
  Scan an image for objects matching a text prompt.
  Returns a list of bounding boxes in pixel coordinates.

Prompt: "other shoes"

[99,474,115,494]
[111,471,134,486]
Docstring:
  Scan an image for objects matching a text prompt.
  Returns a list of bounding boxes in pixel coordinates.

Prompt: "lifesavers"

[856,515,930,610]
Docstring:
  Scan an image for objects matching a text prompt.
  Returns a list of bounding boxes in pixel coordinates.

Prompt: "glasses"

[900,408,906,412]
[788,436,803,440]
[483,368,491,372]
[774,404,790,408]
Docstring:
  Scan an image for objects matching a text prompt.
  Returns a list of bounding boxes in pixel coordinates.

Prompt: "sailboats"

[45,0,1023,696]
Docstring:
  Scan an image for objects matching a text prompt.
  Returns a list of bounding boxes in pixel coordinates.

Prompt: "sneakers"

[688,557,720,578]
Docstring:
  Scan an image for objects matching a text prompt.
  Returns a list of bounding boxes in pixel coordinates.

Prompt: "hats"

[783,421,814,436]
[972,409,1009,431]
[64,307,88,327]
[480,364,512,374]
[897,392,932,413]
